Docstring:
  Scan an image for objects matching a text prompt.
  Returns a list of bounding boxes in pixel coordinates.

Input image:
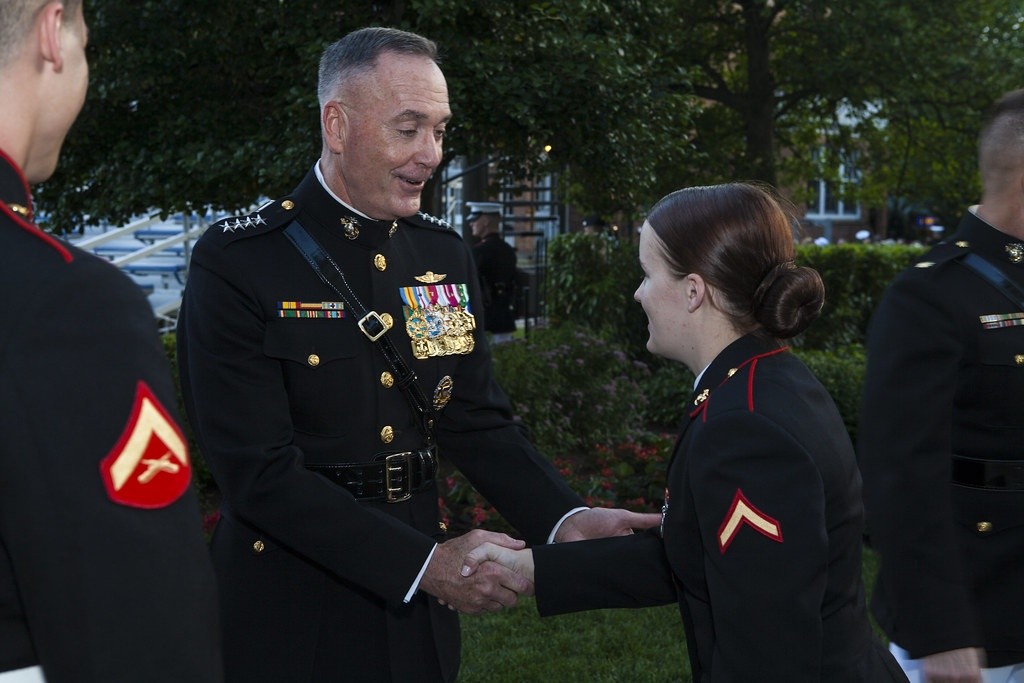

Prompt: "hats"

[466,201,501,220]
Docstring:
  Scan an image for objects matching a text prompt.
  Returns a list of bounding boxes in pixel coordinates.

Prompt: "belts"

[299,442,440,503]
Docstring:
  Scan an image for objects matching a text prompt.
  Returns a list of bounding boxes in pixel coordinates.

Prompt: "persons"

[1,0,227,683]
[847,85,1024,683]
[182,23,667,683]
[436,181,908,682]
[463,199,516,344]
